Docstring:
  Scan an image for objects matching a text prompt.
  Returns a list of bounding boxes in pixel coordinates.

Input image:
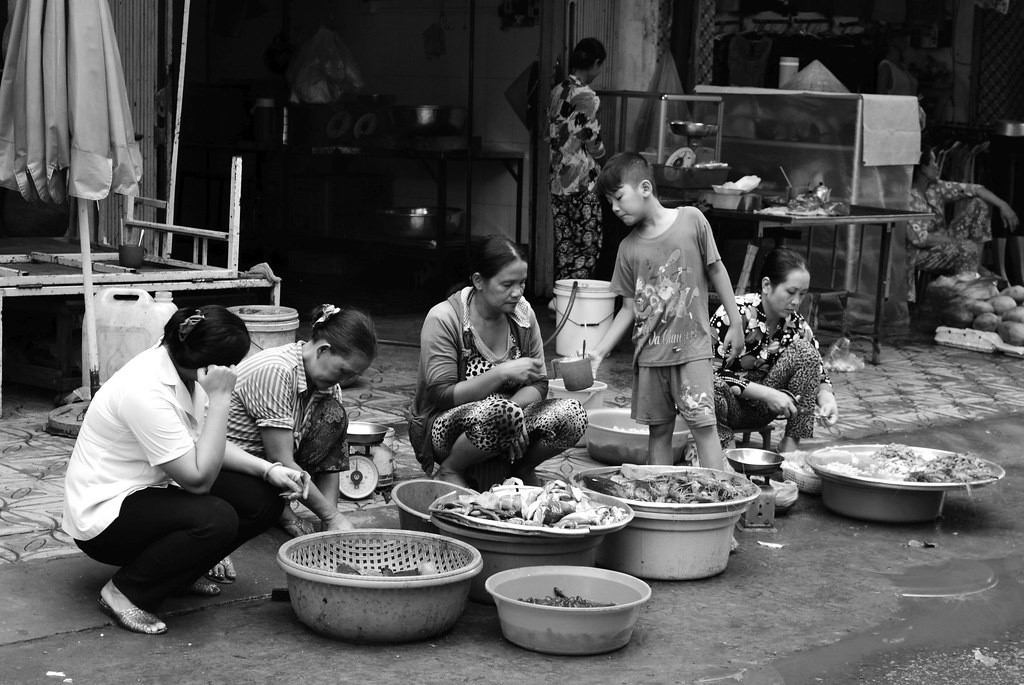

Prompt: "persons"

[409,234,588,488]
[908,143,1020,276]
[207,302,375,588]
[579,151,746,551]
[708,247,838,459]
[875,25,919,95]
[60,305,309,634]
[547,37,607,313]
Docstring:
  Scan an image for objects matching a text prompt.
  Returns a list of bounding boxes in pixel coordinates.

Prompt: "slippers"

[96,595,167,635]
[170,577,221,596]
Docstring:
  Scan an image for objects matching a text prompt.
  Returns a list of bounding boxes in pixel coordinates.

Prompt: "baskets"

[278,528,481,581]
[776,452,822,495]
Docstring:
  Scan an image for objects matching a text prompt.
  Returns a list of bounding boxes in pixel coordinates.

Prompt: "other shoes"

[511,463,546,487]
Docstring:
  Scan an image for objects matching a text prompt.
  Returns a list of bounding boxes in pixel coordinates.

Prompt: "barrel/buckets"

[552,279,620,358]
[546,378,608,448]
[223,304,300,387]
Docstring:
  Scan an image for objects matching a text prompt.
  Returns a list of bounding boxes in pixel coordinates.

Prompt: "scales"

[337,421,388,500]
[660,122,720,186]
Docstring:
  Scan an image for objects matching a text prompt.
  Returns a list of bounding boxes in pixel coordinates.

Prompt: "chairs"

[924,121,995,153]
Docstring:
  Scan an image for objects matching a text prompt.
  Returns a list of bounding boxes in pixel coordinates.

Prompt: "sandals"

[274,509,316,537]
[978,267,1001,280]
[205,554,236,584]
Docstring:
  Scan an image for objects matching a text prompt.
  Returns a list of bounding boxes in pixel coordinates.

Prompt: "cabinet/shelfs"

[237,141,473,302]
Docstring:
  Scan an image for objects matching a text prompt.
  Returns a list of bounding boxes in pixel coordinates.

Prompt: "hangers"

[742,17,857,44]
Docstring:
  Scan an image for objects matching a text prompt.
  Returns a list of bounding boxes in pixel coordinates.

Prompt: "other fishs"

[582,475,624,499]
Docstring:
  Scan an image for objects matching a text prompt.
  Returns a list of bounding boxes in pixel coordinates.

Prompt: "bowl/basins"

[585,408,692,466]
[652,162,732,191]
[274,551,483,642]
[812,469,948,523]
[391,478,481,536]
[430,513,608,607]
[485,565,652,654]
[383,104,467,136]
[606,502,755,580]
[380,206,464,239]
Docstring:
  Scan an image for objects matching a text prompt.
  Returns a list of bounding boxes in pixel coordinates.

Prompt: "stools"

[733,428,774,450]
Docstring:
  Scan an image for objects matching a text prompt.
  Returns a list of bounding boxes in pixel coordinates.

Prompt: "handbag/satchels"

[286,27,361,104]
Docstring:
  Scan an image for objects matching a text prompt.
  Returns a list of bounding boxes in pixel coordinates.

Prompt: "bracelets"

[263,462,282,480]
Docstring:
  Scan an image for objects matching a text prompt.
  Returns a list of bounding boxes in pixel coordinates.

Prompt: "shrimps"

[614,472,753,503]
[904,451,998,482]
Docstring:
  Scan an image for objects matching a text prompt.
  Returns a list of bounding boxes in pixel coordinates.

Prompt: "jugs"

[812,181,832,204]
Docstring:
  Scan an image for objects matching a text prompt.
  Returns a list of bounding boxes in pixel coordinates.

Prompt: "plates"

[726,447,785,474]
[670,119,718,138]
[346,421,389,443]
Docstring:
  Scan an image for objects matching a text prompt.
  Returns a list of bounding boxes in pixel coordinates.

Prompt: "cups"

[254,99,276,141]
[550,355,594,392]
[778,56,799,90]
[119,243,148,268]
[786,186,809,205]
